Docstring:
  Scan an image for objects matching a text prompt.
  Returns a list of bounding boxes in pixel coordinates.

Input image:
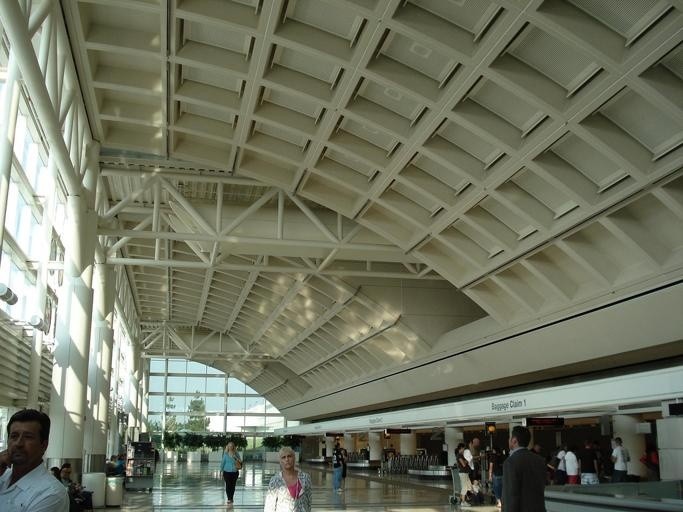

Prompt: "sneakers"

[227,499,234,504]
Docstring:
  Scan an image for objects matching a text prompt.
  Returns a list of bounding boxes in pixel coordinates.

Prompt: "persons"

[341,448,348,491]
[219,441,243,505]
[453,424,631,512]
[389,443,394,449]
[0,409,70,511]
[264,447,313,512]
[51,448,159,512]
[332,441,345,492]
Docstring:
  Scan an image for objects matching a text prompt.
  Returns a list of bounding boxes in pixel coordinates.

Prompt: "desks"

[125,474,153,496]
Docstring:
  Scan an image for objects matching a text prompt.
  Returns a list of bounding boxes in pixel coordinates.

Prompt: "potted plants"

[181,432,207,462]
[258,437,284,463]
[204,433,227,462]
[279,434,306,462]
[162,431,183,461]
[225,434,248,462]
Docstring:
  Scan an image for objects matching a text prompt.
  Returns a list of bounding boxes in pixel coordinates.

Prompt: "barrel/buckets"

[81,472,106,508]
[105,477,125,506]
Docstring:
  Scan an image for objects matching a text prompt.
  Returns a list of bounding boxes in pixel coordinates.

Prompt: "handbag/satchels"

[235,459,242,469]
